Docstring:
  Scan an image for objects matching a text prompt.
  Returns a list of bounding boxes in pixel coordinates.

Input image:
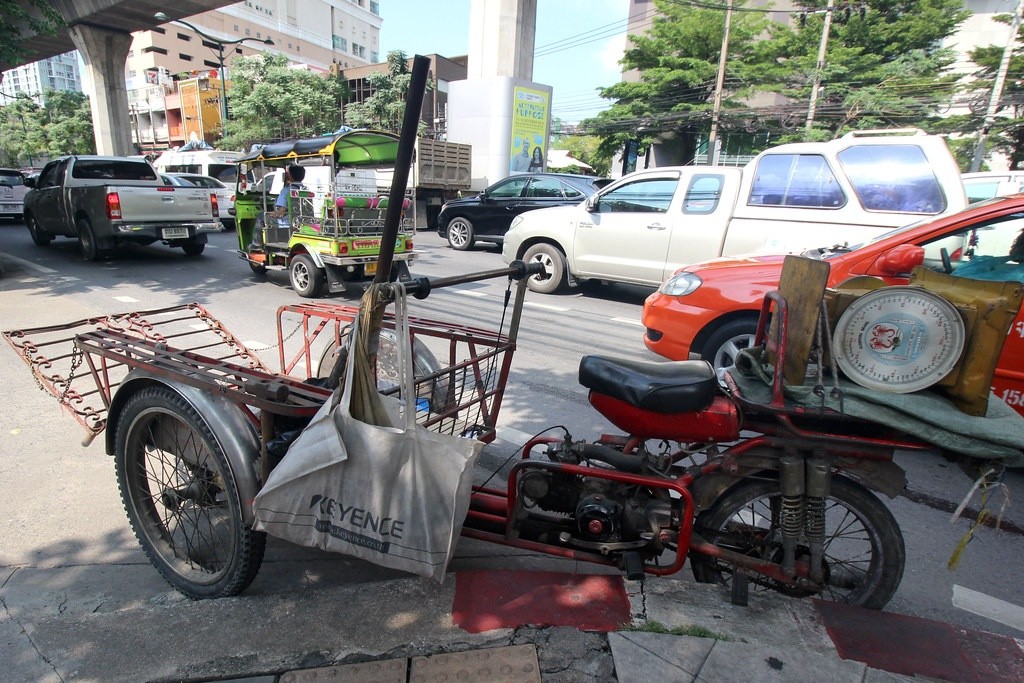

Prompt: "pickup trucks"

[21,154,225,263]
[500,126,969,296]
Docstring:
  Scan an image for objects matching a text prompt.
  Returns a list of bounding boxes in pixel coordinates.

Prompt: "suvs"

[436,171,616,251]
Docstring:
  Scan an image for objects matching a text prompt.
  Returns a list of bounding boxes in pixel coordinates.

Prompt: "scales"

[773,253,1024,418]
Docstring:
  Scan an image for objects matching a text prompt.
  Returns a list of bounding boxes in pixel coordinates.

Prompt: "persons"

[950,227,1024,288]
[512,138,531,172]
[249,163,309,250]
[528,147,543,173]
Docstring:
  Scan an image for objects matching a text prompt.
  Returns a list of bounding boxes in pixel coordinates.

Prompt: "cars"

[959,169,1024,204]
[640,191,1024,419]
[0,167,35,223]
[18,166,44,186]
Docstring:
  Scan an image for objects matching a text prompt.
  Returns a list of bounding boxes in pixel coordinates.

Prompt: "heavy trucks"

[375,136,473,230]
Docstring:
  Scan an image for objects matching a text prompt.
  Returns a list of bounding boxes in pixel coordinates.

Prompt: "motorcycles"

[227,128,421,299]
[0,299,1006,611]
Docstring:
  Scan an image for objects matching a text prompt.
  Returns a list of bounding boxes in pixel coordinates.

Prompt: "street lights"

[0,91,43,167]
[153,11,276,137]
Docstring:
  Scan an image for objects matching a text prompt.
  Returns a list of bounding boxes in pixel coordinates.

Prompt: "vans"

[152,150,247,234]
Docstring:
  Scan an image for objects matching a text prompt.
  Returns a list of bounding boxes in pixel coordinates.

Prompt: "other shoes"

[247,244,262,251]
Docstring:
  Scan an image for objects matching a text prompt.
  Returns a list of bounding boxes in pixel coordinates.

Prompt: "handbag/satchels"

[251,280,485,583]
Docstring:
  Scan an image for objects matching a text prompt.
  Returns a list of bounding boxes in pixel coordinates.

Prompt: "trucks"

[255,164,378,220]
